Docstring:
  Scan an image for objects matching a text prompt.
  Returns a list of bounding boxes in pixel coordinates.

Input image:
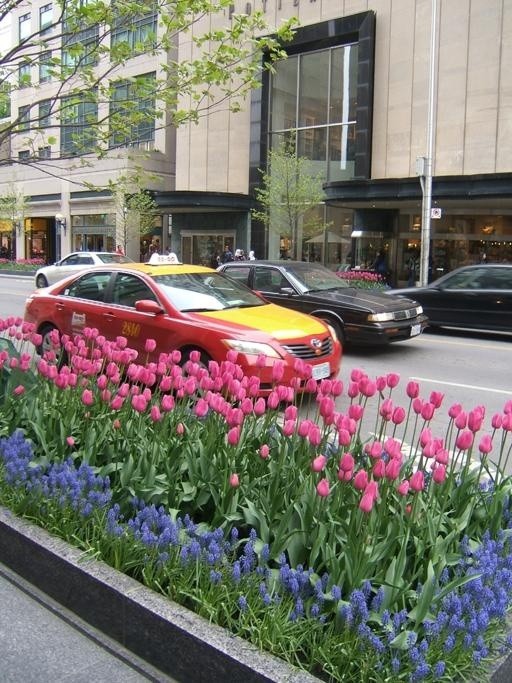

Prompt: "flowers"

[334,271,388,290]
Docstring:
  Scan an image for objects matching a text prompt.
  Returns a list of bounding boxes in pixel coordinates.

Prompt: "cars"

[21,252,343,396]
[34,250,136,288]
[383,263,512,332]
[205,259,430,350]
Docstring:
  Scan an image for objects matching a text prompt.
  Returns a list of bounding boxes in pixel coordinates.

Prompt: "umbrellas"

[305,232,351,263]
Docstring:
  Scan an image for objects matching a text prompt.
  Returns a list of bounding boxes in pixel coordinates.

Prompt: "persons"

[218,246,257,266]
[0,244,8,258]
[115,245,125,256]
[139,239,171,263]
[367,249,389,287]
[407,255,416,288]
